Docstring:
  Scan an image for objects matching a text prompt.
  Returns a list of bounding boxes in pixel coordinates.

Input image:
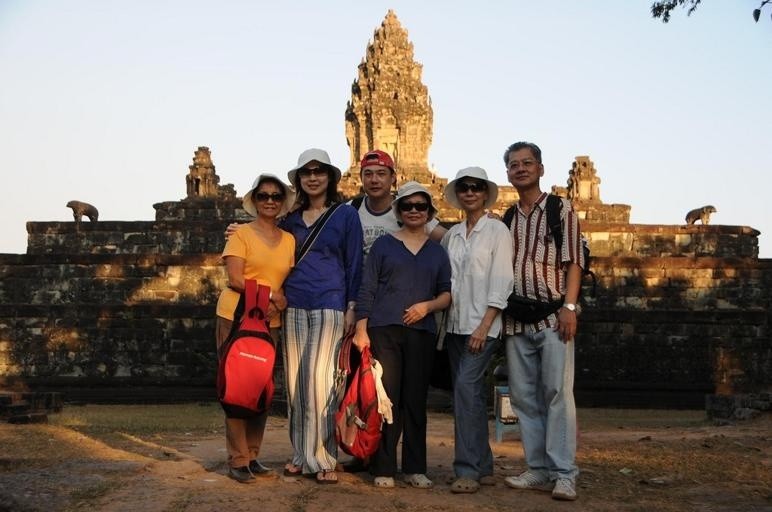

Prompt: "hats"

[360,150,398,190]
[241,170,297,222]
[288,149,342,190]
[444,166,500,211]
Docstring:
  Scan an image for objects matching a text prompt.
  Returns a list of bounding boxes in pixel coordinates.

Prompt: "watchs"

[563,302,578,312]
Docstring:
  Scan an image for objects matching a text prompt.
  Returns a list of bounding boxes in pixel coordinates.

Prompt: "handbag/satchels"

[504,294,562,326]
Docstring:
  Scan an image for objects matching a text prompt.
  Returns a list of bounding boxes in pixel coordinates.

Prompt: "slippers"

[401,470,434,490]
[284,461,303,478]
[374,475,396,490]
[480,474,498,488]
[450,473,481,495]
[317,467,340,484]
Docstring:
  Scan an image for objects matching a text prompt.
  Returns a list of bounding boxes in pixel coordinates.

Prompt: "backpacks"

[502,193,598,316]
[332,332,392,461]
[217,279,275,422]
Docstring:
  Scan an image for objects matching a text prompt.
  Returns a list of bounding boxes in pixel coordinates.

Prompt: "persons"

[224,149,364,484]
[214,172,295,484]
[438,166,515,494]
[348,180,453,490]
[498,141,586,500]
[342,148,449,474]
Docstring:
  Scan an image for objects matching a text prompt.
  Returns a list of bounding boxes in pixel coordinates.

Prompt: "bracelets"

[347,302,356,310]
[270,291,273,301]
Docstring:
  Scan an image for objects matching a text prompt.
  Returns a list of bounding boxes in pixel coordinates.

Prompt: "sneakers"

[551,473,578,502]
[504,470,550,491]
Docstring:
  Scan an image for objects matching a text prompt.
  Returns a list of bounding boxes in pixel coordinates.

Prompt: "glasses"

[389,180,439,225]
[298,167,329,177]
[455,182,488,194]
[506,158,542,171]
[398,201,429,213]
[251,189,285,205]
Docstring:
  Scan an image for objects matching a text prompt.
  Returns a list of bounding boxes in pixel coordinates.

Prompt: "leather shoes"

[251,459,275,478]
[229,464,257,485]
